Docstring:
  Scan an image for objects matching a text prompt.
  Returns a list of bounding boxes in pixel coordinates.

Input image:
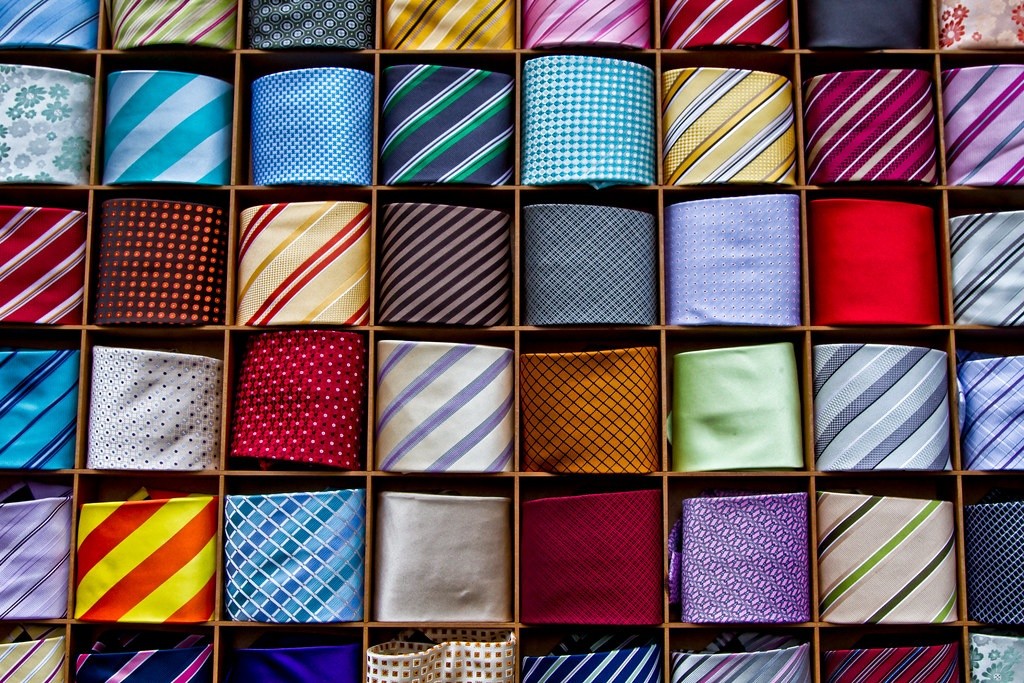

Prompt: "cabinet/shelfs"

[0,0,1024,683]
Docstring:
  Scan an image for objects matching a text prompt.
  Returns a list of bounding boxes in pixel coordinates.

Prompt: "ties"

[0,0,1024,683]
